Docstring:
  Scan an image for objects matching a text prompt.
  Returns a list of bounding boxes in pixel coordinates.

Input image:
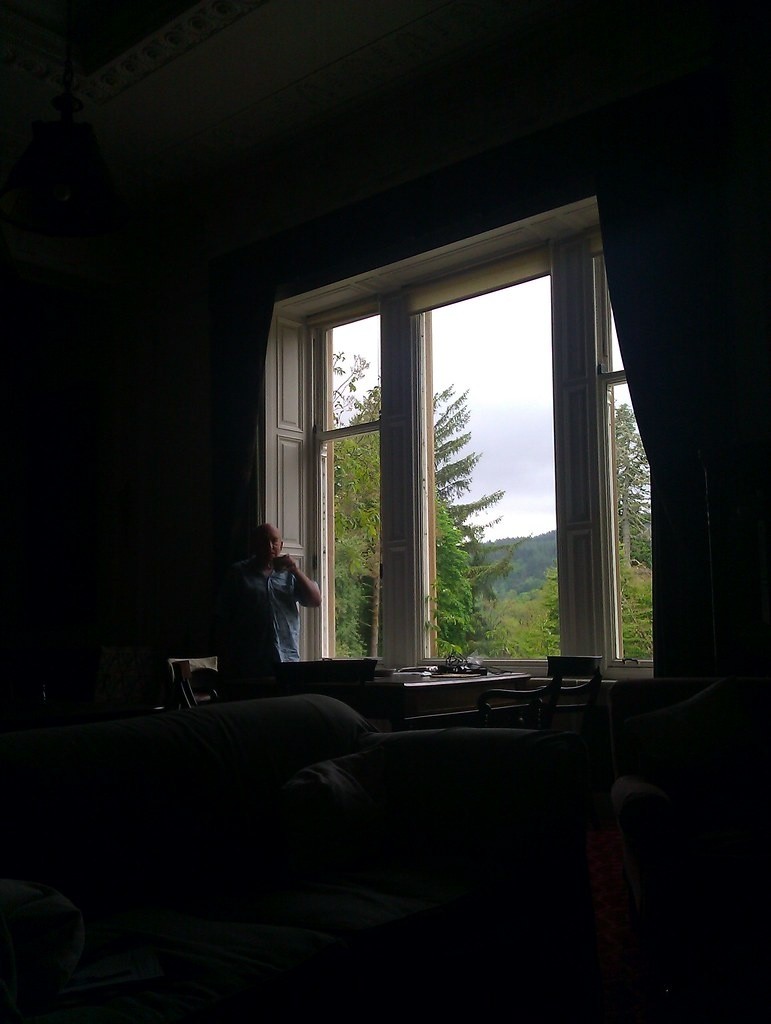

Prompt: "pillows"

[626,678,743,759]
[275,742,396,856]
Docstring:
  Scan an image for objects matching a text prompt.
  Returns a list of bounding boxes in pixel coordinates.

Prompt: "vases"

[547,656,603,678]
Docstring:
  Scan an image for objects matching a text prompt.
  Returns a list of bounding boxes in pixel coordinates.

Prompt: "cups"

[273,557,286,573]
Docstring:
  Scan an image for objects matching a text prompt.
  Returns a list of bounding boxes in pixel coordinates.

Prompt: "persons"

[214,523,322,698]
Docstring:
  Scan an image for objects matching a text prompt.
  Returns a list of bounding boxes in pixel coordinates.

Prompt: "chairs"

[479,672,603,736]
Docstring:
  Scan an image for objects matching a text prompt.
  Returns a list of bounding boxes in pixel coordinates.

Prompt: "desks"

[220,677,530,713]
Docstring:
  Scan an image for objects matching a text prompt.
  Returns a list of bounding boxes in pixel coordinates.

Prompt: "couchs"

[607,678,771,1024]
[1,690,599,1023]
